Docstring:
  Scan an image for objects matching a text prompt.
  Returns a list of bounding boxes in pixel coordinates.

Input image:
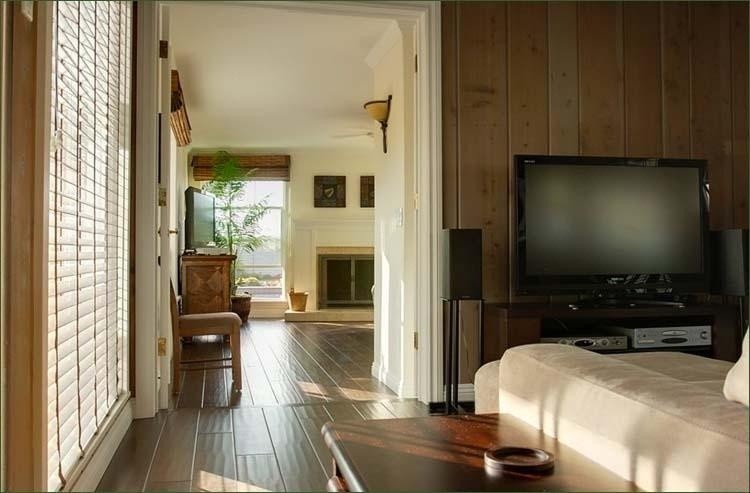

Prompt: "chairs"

[158,254,244,398]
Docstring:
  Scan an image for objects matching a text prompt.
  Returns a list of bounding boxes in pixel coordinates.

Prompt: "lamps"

[364,95,393,154]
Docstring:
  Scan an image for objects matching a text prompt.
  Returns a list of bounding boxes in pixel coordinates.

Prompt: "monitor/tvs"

[509,154,710,308]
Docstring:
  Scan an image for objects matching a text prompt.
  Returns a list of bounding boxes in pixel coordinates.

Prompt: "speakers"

[710,228,748,297]
[439,228,482,301]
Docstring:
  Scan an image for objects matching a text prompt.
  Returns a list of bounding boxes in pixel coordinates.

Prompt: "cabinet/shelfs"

[486,303,742,363]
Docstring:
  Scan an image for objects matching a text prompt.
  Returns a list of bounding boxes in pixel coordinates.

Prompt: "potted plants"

[204,149,275,322]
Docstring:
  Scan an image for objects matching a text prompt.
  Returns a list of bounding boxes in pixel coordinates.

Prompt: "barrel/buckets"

[231,294,251,322]
[288,287,308,311]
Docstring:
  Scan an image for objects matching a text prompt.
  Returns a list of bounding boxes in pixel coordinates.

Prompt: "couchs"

[473,349,750,493]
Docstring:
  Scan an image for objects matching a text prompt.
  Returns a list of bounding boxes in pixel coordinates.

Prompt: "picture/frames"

[313,175,346,209]
